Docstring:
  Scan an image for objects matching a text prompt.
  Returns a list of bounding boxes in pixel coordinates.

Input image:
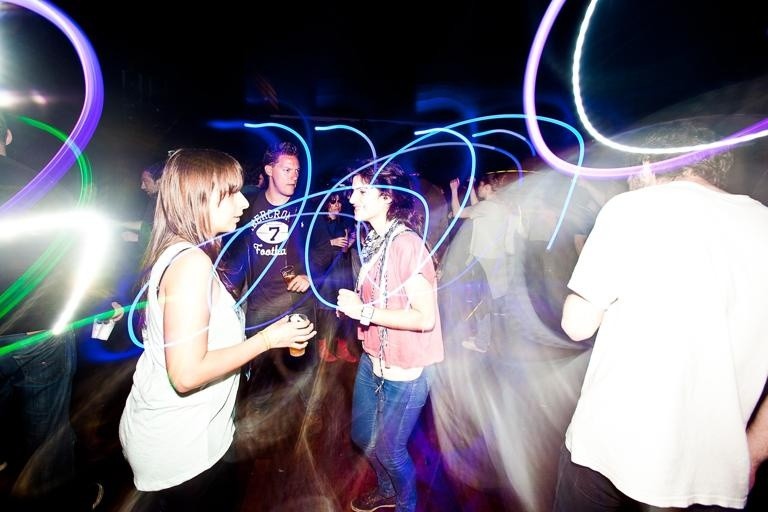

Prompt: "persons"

[1,119,768,511]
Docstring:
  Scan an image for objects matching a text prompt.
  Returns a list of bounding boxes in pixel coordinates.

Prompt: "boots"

[317,337,359,363]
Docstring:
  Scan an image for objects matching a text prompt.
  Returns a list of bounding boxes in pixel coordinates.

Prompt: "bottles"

[342,228,350,253]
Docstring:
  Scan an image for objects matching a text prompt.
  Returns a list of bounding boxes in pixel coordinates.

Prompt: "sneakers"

[351,488,398,512]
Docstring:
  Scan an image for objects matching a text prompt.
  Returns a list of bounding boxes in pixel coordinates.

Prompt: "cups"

[280,264,298,290]
[287,313,307,356]
